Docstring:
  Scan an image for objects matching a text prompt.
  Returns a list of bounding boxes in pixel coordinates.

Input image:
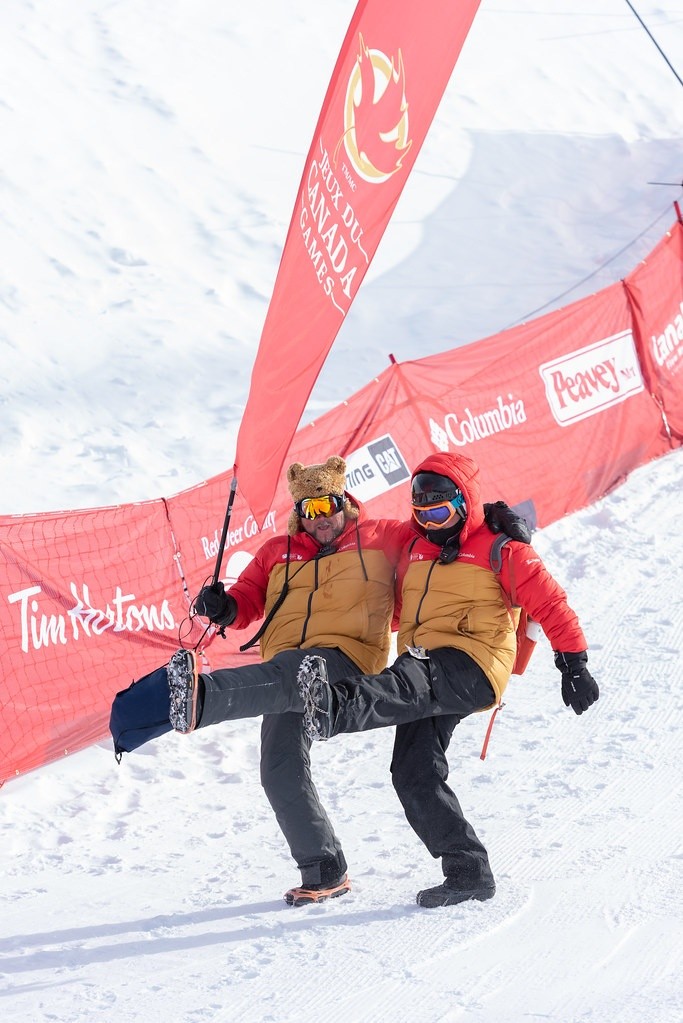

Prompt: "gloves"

[554,651,599,715]
[483,500,531,544]
[195,582,237,627]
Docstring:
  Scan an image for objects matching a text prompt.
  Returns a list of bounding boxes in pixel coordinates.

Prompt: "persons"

[165,457,529,906]
[297,451,599,906]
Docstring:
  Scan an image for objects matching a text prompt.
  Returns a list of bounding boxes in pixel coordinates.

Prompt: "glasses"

[297,495,345,520]
[412,501,456,529]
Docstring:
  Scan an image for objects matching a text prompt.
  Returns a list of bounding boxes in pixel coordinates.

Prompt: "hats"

[287,456,359,537]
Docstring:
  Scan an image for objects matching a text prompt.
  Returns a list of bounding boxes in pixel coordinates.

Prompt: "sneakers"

[166,649,203,734]
[299,656,338,741]
[287,872,349,907]
[416,885,496,908]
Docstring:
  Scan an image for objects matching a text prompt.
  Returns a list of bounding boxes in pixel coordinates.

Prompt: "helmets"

[411,473,467,546]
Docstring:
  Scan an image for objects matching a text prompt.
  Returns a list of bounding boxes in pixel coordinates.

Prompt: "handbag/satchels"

[109,617,214,765]
